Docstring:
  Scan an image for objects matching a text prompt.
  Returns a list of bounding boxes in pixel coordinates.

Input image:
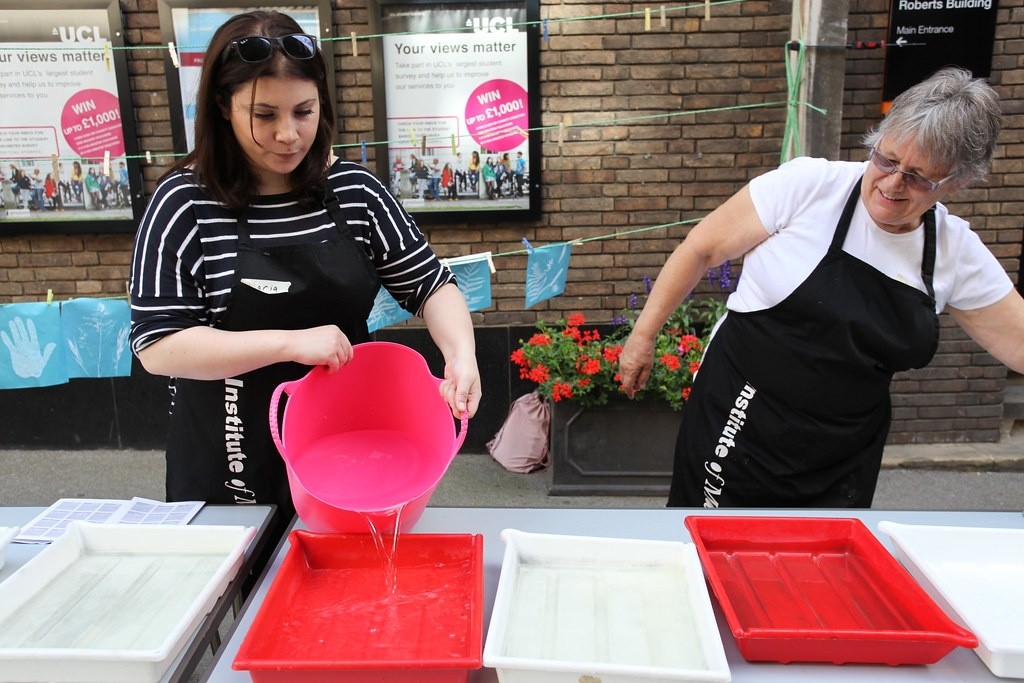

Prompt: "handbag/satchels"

[485,389,550,474]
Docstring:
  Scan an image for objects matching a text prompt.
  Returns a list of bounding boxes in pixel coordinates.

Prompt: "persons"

[619,68,1024,511]
[129,10,482,611]
[391,149,526,201]
[0,160,128,211]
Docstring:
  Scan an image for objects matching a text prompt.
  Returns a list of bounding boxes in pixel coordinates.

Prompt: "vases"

[546,388,681,496]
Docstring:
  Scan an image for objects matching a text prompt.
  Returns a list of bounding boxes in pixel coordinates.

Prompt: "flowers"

[511,258,733,412]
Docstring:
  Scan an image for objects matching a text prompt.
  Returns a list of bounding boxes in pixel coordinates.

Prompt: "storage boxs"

[684,515,978,668]
[876,520,1024,680]
[232,532,486,683]
[0,520,258,683]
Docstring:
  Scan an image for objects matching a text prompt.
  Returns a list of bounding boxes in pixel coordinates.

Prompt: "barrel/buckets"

[271,342,469,534]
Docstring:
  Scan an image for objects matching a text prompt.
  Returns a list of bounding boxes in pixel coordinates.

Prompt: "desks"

[0,505,277,682]
[201,504,1024,683]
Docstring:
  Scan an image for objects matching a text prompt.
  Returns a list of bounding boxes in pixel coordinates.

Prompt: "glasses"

[868,133,961,193]
[220,33,317,67]
